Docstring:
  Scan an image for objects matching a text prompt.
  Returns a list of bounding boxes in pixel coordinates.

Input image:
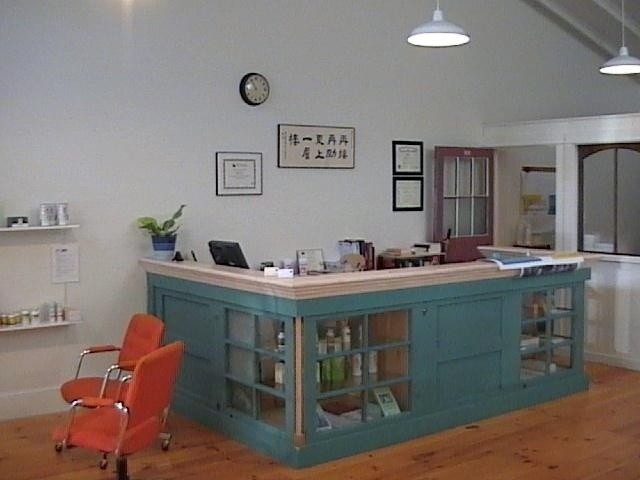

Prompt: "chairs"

[52,312,184,480]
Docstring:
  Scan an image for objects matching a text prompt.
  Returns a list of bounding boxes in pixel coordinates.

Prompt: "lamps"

[599,0,640,74]
[406,0,471,48]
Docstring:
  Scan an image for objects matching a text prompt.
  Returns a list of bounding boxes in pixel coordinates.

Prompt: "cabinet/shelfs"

[0,224,83,331]
[379,253,446,270]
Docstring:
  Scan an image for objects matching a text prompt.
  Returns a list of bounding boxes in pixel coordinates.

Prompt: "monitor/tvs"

[209,241,249,269]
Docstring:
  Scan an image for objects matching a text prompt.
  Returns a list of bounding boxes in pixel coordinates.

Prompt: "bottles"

[39,199,70,226]
[276,321,364,357]
[0,302,66,328]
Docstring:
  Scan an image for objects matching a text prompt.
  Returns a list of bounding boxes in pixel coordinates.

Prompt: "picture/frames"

[393,140,425,212]
[215,151,263,196]
[277,124,356,170]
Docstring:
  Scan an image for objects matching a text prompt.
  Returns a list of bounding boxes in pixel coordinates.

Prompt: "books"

[519,334,540,350]
[338,239,443,272]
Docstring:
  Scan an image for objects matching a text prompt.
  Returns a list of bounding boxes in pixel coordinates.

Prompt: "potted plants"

[137,205,186,260]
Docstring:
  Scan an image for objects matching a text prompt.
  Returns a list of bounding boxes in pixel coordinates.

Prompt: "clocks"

[240,73,269,106]
